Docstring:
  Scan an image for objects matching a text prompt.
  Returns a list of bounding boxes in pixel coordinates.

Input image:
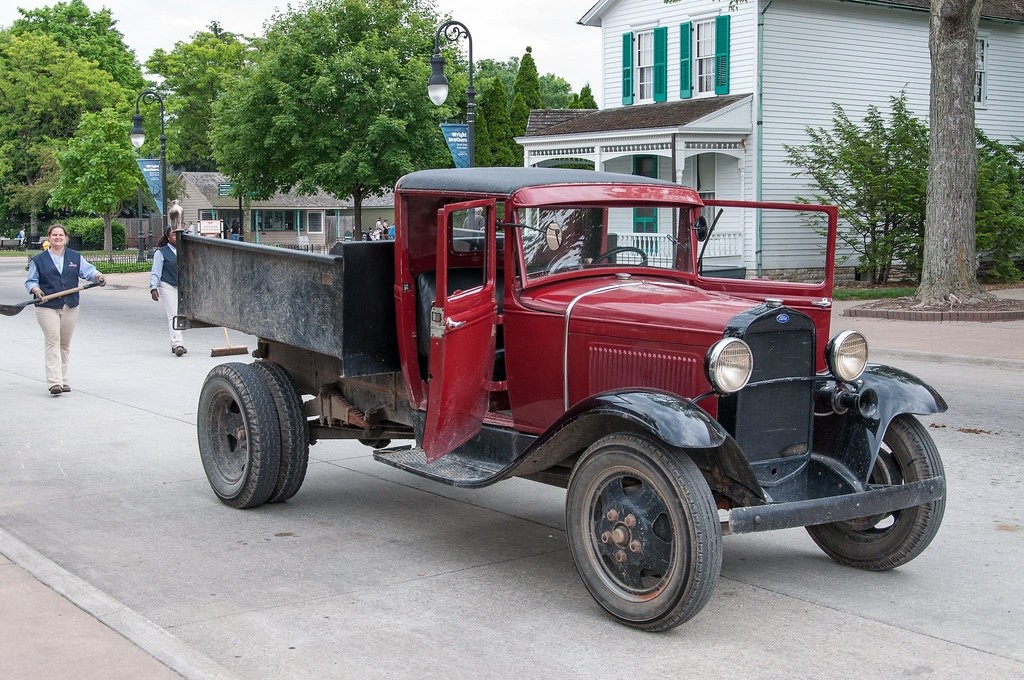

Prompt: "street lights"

[130,91,168,235]
[426,20,475,166]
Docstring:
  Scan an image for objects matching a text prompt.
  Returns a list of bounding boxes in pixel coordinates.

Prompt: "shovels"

[0,281,103,316]
[210,327,247,357]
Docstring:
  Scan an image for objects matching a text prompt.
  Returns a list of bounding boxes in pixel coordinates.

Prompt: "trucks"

[172,164,949,633]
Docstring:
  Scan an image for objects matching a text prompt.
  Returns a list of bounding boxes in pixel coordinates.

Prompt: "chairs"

[414,266,506,385]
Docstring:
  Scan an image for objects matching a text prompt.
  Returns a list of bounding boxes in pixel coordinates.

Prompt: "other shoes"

[62,385,71,392]
[50,385,63,395]
[171,346,188,354]
[175,346,184,357]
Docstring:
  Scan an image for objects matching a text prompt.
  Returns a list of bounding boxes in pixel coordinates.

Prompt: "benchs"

[31,237,51,250]
[0,240,21,252]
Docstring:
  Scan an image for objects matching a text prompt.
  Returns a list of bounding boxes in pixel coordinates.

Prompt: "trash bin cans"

[68,234,82,250]
[25,235,40,250]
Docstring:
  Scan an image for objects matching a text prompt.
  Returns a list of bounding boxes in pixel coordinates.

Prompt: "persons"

[366,219,395,241]
[25,225,106,394]
[149,225,187,355]
[220,219,240,241]
[183,221,195,235]
[19,228,26,246]
[462,206,485,230]
